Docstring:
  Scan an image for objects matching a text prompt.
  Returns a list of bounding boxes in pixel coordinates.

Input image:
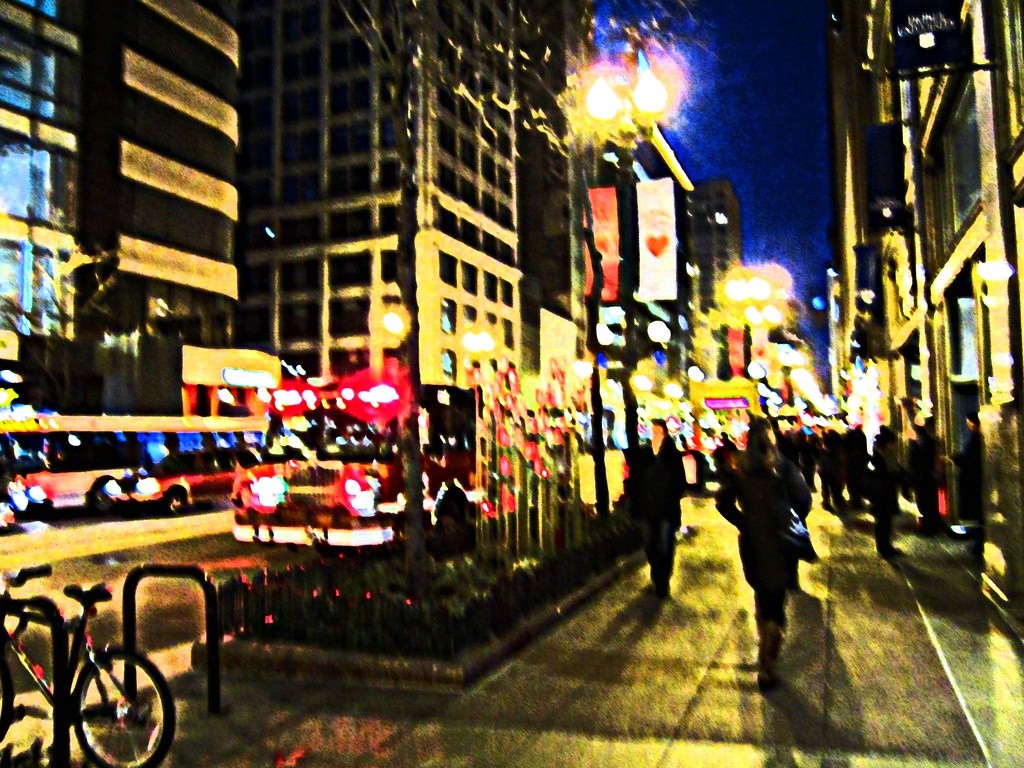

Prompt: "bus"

[232,380,576,561]
[0,412,317,512]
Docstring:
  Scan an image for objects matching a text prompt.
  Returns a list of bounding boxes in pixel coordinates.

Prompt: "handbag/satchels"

[780,510,818,564]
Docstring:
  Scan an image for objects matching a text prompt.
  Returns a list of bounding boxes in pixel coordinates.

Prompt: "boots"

[756,623,779,691]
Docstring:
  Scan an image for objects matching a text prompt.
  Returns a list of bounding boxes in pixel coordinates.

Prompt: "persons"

[715,421,946,621]
[714,415,811,694]
[950,416,988,542]
[627,419,686,586]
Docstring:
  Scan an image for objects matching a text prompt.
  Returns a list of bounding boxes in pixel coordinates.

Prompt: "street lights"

[725,270,772,377]
[590,72,674,516]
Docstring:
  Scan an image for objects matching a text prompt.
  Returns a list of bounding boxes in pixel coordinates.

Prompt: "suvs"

[104,448,262,516]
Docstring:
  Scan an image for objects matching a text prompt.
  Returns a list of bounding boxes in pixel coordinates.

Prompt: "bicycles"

[0,564,175,767]
